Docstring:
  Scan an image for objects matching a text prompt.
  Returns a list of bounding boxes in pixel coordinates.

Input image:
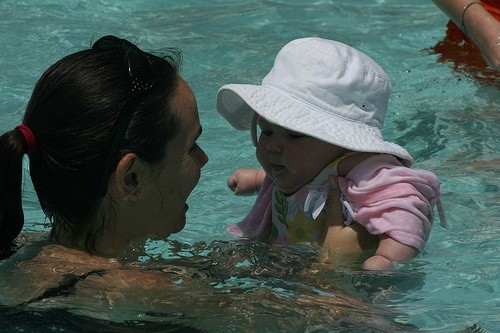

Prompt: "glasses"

[89,35,157,197]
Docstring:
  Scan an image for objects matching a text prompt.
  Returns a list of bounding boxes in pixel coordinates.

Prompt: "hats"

[217,36,414,167]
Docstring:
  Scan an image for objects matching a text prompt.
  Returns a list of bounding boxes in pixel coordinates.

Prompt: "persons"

[432,0,500,71]
[218,37,446,273]
[0,35,419,333]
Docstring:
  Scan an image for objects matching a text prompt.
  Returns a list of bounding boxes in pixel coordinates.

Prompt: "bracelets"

[461,2,483,34]
